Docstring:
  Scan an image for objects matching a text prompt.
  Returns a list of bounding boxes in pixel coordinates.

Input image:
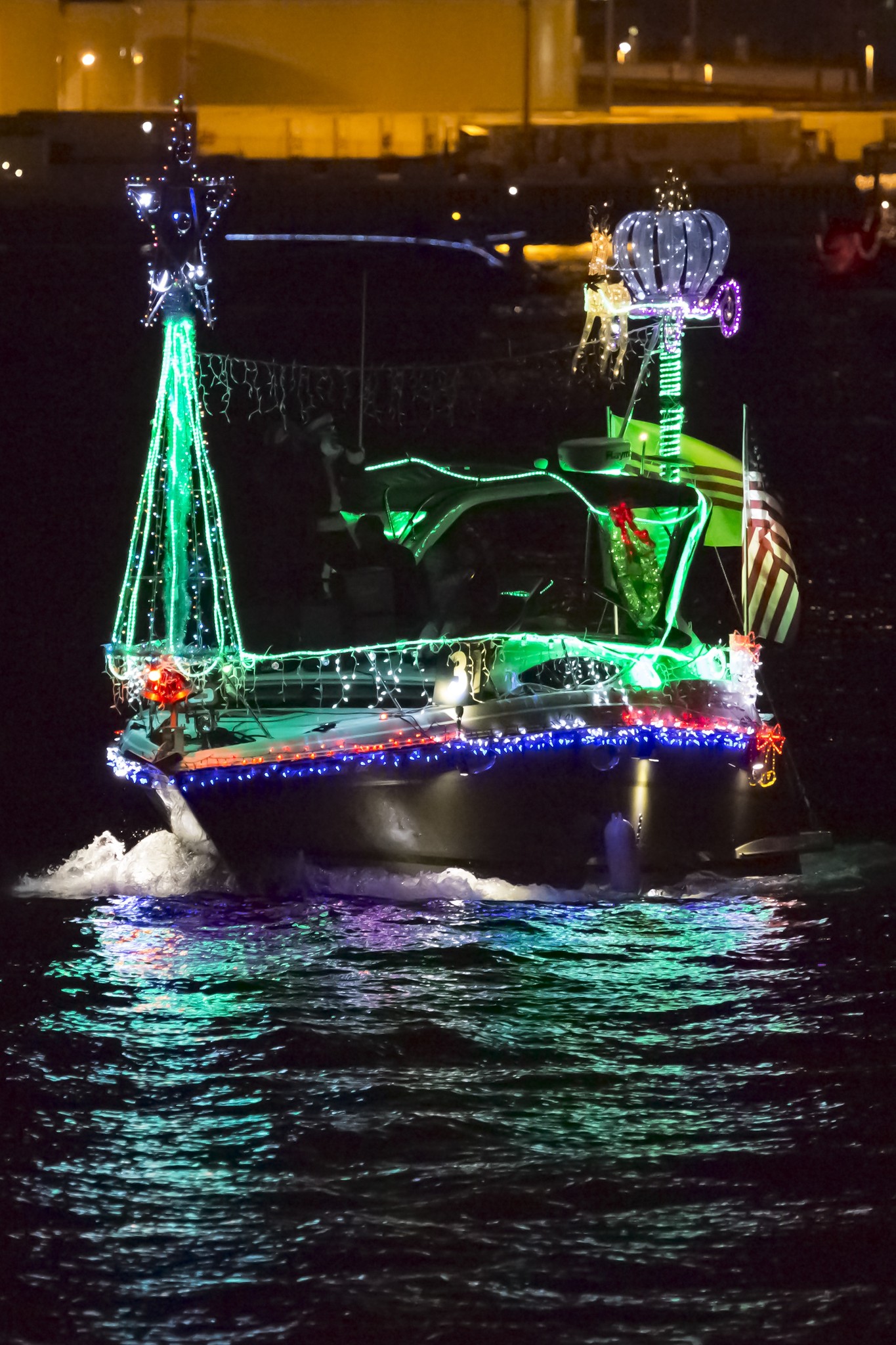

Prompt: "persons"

[353,515,431,626]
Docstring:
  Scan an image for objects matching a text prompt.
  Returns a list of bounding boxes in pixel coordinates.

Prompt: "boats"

[115,87,812,894]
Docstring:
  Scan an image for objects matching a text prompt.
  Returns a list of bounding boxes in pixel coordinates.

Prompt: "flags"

[743,421,801,643]
